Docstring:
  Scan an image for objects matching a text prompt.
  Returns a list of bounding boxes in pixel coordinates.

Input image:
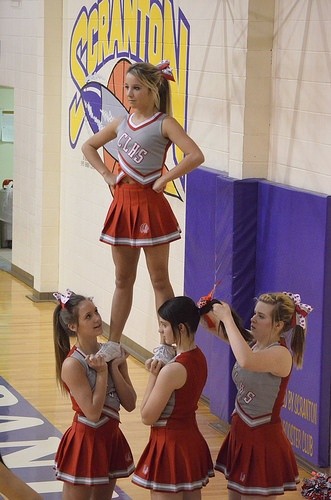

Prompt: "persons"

[53,291,138,500]
[81,61,205,345]
[129,296,253,500]
[200,291,312,500]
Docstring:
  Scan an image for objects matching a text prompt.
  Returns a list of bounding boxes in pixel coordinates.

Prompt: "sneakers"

[89,341,121,362]
[145,344,176,367]
